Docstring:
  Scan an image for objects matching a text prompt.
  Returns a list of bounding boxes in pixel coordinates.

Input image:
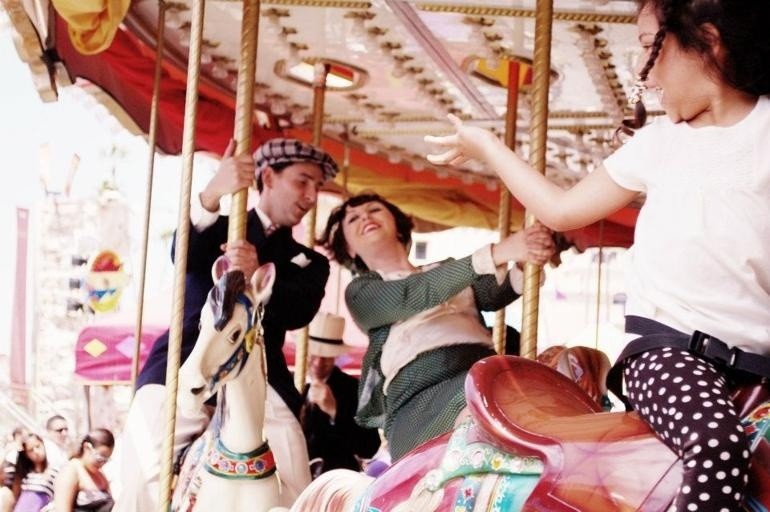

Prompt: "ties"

[264,225,276,237]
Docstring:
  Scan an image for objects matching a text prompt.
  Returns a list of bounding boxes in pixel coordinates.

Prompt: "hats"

[308,312,354,357]
[252,138,340,181]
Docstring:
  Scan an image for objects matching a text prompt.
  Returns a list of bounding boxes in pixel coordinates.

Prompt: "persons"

[0,424,31,492]
[109,135,339,512]
[41,415,69,467]
[4,432,53,511]
[53,429,116,512]
[316,188,560,465]
[418,2,770,512]
[302,308,382,480]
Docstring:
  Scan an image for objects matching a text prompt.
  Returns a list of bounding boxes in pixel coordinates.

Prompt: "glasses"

[49,428,69,433]
[88,442,112,464]
